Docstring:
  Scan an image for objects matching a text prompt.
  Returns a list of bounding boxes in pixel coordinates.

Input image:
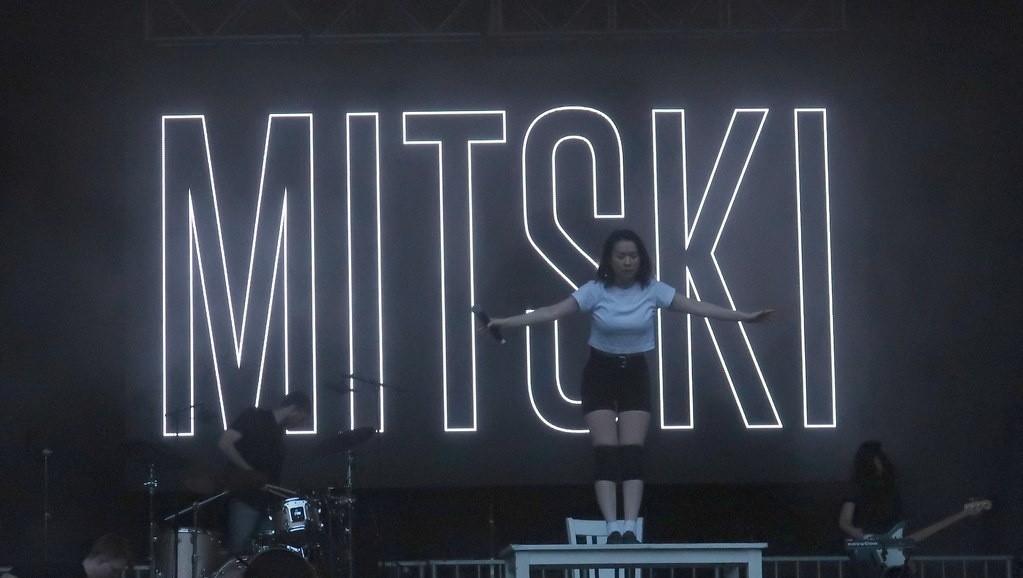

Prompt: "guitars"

[844,495,992,578]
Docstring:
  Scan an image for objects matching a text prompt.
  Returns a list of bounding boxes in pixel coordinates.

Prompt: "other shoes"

[607,531,624,544]
[623,531,640,544]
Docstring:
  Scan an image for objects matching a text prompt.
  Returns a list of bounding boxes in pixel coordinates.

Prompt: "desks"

[500,543,768,578]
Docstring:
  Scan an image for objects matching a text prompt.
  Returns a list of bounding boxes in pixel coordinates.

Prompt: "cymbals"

[113,441,188,469]
[311,428,375,456]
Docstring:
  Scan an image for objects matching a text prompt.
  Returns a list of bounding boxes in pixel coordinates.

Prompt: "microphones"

[470,305,506,345]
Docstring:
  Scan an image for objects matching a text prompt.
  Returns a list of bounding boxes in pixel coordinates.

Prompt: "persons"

[217,392,312,555]
[54,533,129,578]
[837,440,921,578]
[478,229,775,544]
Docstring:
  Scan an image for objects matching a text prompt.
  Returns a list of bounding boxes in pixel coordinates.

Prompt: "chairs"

[566,517,642,578]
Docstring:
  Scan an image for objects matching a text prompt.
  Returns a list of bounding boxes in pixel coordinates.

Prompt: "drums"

[266,493,322,543]
[204,543,320,578]
[153,527,222,578]
